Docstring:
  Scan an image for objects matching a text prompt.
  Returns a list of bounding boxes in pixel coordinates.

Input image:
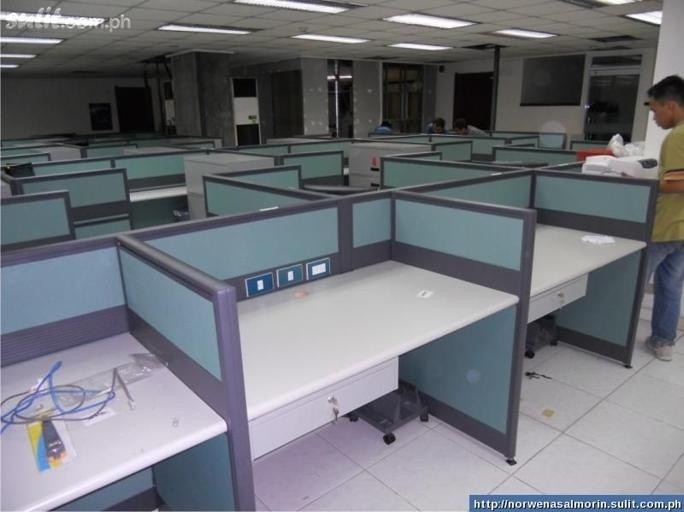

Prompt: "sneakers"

[644,335,674,361]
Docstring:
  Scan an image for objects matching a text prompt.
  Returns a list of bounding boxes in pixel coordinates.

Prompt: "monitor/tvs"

[6,162,35,177]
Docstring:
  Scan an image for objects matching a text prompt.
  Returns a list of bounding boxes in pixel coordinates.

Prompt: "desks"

[0,132,659,510]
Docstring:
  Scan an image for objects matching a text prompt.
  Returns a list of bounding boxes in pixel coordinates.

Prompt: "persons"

[374,120,394,135]
[425,118,450,135]
[453,118,488,136]
[646,75,684,362]
